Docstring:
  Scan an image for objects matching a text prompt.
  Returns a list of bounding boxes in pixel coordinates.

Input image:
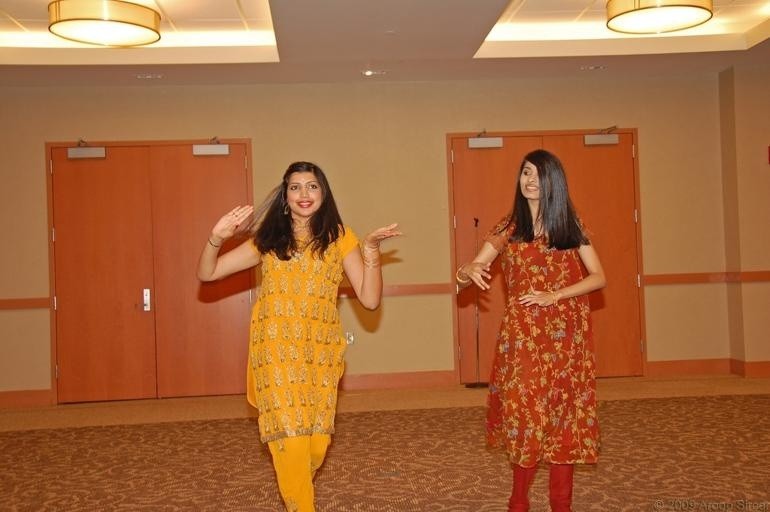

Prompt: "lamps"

[44,1,166,48]
[603,0,714,39]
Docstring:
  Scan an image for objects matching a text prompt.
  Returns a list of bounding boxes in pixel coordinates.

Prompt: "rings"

[233,213,238,218]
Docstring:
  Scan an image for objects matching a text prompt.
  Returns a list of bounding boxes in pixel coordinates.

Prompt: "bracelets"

[362,241,382,269]
[455,263,471,284]
[549,291,559,308]
[207,238,221,249]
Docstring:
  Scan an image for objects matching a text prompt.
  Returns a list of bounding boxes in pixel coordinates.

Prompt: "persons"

[453,149,608,510]
[196,160,403,512]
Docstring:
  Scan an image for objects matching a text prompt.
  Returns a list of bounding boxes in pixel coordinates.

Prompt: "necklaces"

[294,223,308,229]
